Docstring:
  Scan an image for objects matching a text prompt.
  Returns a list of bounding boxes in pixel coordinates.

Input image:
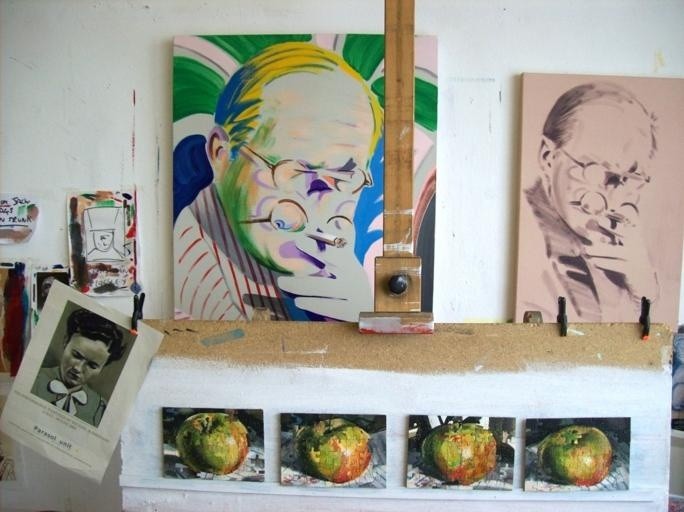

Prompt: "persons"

[30,308,126,428]
[174,41,384,323]
[515,83,661,322]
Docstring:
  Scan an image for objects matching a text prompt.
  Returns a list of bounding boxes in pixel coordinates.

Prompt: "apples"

[541,424,612,486]
[176,412,249,475]
[298,414,373,483]
[422,422,498,486]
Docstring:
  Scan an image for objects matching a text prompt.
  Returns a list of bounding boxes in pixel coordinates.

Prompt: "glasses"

[562,150,650,190]
[245,145,374,197]
[237,201,355,247]
[571,192,642,223]
